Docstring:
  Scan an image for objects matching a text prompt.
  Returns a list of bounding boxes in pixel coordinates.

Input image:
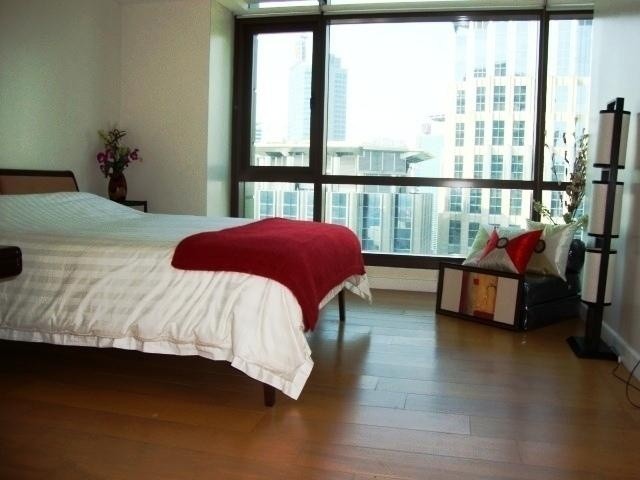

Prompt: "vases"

[107,171,127,202]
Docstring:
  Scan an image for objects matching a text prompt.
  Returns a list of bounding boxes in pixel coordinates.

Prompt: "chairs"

[435,230,586,332]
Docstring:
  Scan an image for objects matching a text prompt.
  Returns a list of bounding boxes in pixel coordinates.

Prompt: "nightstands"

[117,200,148,213]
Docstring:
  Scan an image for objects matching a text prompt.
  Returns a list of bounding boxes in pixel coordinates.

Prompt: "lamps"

[567,96,632,359]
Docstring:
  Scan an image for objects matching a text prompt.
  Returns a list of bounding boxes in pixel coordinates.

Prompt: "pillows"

[524,217,577,282]
[461,221,545,273]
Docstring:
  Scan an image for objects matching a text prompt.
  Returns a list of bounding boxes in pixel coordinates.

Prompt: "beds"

[1,166,371,405]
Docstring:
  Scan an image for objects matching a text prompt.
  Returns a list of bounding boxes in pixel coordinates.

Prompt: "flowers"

[96,127,139,177]
